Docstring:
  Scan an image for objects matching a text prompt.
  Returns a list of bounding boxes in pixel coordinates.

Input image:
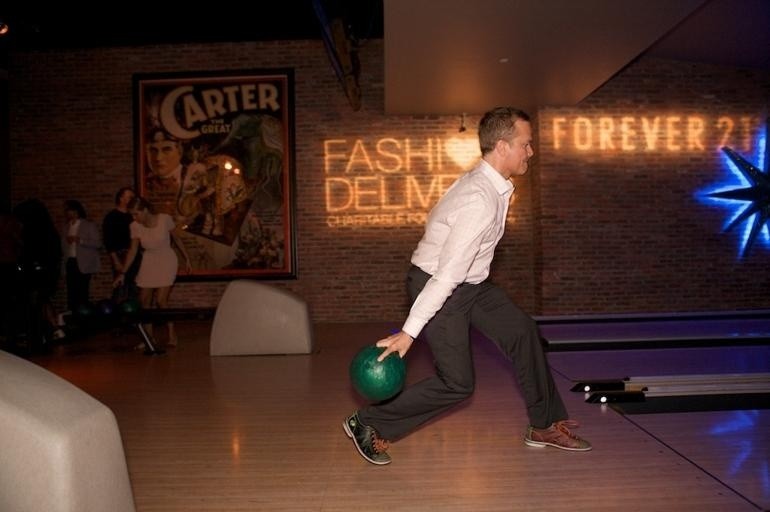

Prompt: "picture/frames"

[130,66,299,283]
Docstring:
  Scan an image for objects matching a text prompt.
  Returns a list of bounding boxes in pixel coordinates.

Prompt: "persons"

[102,186,143,334]
[112,195,193,350]
[144,126,205,232]
[343,106,595,466]
[61,199,101,311]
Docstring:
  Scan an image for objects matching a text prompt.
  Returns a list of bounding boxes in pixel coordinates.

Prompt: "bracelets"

[119,271,126,275]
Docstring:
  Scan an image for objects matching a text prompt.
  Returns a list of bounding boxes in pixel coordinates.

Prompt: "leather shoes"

[342,409,392,465]
[524,422,592,452]
